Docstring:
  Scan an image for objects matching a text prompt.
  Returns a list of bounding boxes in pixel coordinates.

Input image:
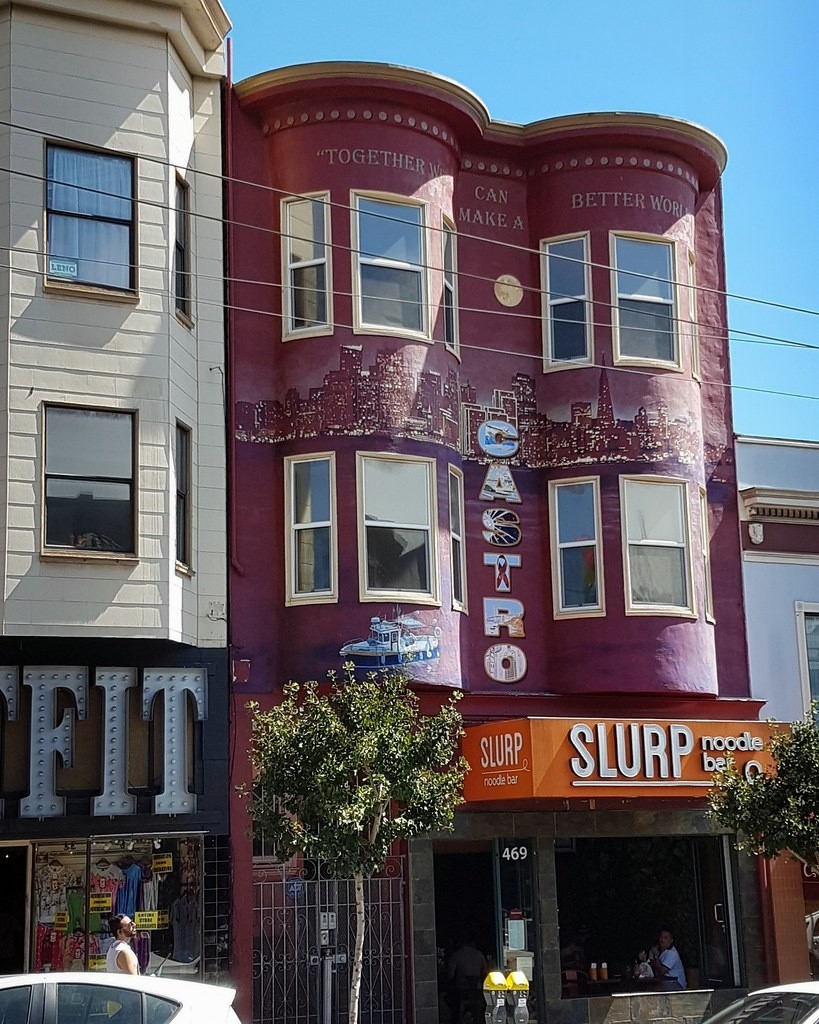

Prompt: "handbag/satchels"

[633,960,654,978]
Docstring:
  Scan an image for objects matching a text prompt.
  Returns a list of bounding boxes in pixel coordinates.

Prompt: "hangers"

[116,853,132,866]
[138,850,152,865]
[48,857,63,868]
[95,852,110,869]
[72,920,87,936]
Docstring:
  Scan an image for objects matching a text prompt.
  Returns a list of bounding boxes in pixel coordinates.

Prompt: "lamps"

[154,838,161,849]
[127,841,135,850]
[63,843,113,851]
[120,840,125,849]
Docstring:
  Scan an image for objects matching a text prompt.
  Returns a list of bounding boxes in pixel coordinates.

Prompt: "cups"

[598,968,608,980]
[587,968,597,982]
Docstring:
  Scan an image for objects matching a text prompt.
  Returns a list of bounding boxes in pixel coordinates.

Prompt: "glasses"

[125,919,133,928]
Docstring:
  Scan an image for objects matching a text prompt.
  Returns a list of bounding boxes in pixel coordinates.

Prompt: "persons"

[560,923,690,991]
[446,936,487,1024]
[106,914,140,975]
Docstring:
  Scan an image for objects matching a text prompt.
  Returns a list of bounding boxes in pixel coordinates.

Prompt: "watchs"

[654,956,658,958]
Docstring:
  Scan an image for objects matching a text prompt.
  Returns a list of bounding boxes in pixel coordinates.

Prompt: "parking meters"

[482,971,509,1024]
[503,971,530,1024]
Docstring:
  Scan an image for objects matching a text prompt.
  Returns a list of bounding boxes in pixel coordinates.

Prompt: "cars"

[704,981,819,1024]
[0,969,243,1024]
